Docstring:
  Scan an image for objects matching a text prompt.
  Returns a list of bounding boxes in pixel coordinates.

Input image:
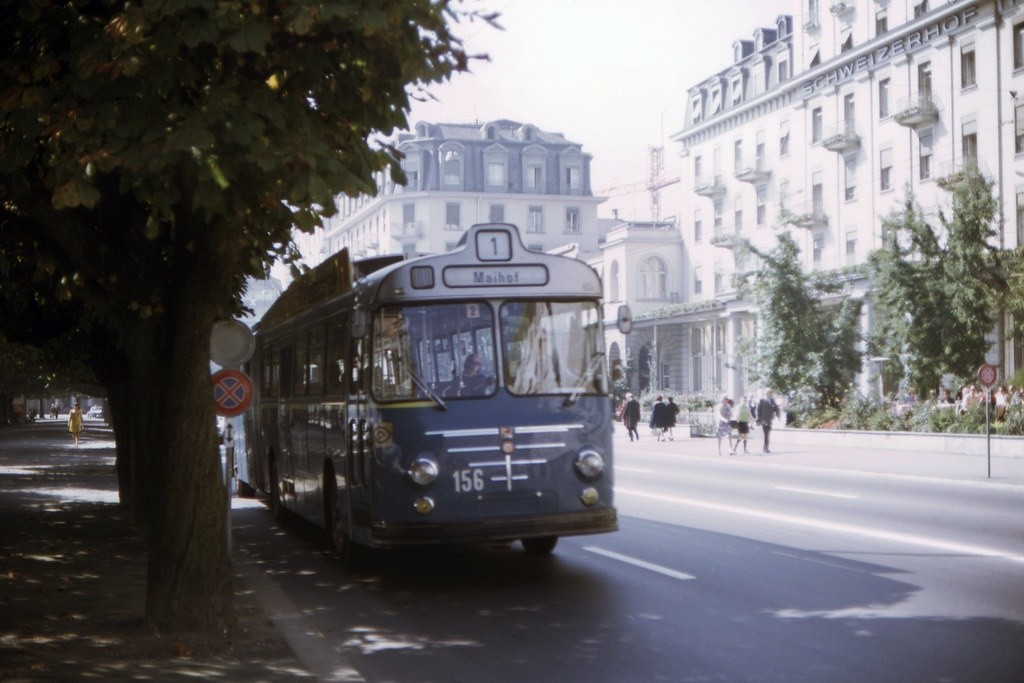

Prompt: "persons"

[623,394,641,442]
[758,388,781,454]
[665,396,680,441]
[730,396,751,454]
[448,352,492,397]
[649,396,666,442]
[618,393,631,436]
[55,403,60,420]
[716,396,737,455]
[50,404,56,420]
[514,328,572,394]
[67,402,85,448]
[28,408,36,423]
[892,379,1024,418]
[749,393,758,429]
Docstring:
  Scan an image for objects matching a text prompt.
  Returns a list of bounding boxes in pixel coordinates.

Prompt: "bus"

[223,218,620,570]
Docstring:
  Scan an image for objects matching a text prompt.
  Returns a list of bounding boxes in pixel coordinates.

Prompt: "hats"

[466,354,483,367]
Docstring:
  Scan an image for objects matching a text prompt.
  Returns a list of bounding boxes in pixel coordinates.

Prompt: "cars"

[86,406,104,420]
